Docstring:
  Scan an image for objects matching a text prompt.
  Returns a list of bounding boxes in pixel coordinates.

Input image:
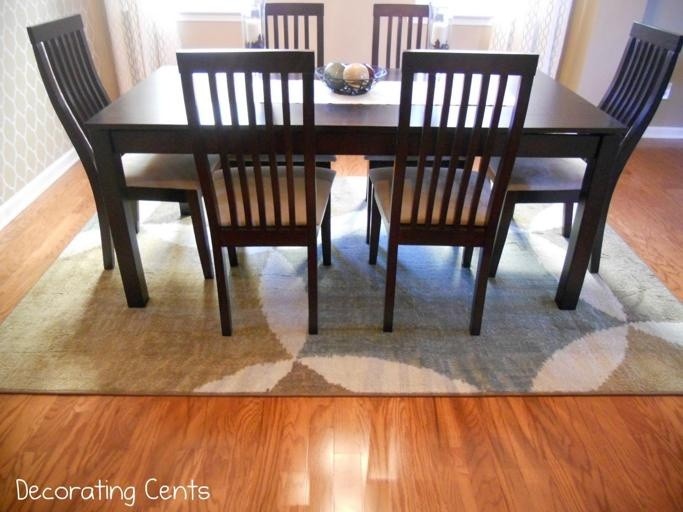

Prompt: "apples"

[324,62,375,88]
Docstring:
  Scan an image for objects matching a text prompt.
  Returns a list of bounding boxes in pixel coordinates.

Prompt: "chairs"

[365,47,539,337]
[365,2,469,246]
[25,11,222,281]
[485,17,682,280]
[221,0,332,269]
[175,47,336,337]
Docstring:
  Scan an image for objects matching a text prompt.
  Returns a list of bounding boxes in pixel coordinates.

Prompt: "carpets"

[1,171,683,398]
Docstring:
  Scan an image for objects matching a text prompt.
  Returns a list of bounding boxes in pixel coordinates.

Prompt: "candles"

[429,19,446,47]
[242,15,261,42]
